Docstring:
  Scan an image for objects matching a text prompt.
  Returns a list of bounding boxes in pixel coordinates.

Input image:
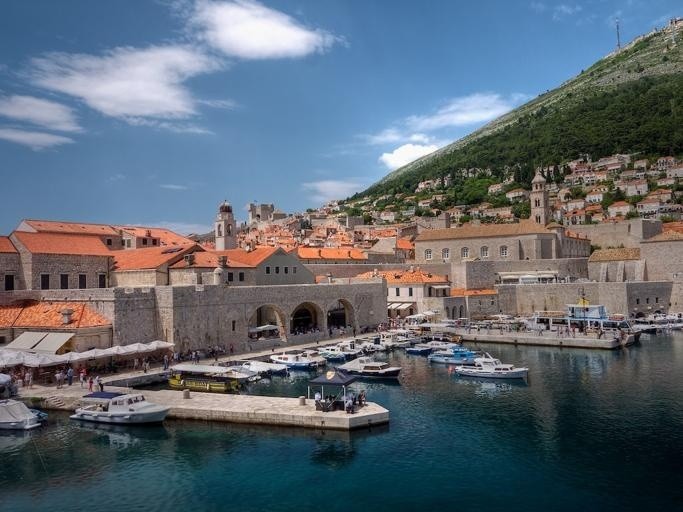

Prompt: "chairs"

[358,392,366,405]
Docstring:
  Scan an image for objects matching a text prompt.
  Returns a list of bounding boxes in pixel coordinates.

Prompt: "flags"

[578,298,590,306]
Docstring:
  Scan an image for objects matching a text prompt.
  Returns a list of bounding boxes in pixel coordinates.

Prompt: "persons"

[313,389,366,414]
[111,343,233,373]
[0,364,102,394]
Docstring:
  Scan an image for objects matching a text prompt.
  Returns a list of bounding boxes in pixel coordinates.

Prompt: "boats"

[270,330,530,379]
[69,393,172,425]
[2,397,50,430]
[631,312,683,335]
[168,360,291,394]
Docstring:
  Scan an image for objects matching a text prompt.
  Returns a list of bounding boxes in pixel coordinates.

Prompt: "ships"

[445,308,636,349]
